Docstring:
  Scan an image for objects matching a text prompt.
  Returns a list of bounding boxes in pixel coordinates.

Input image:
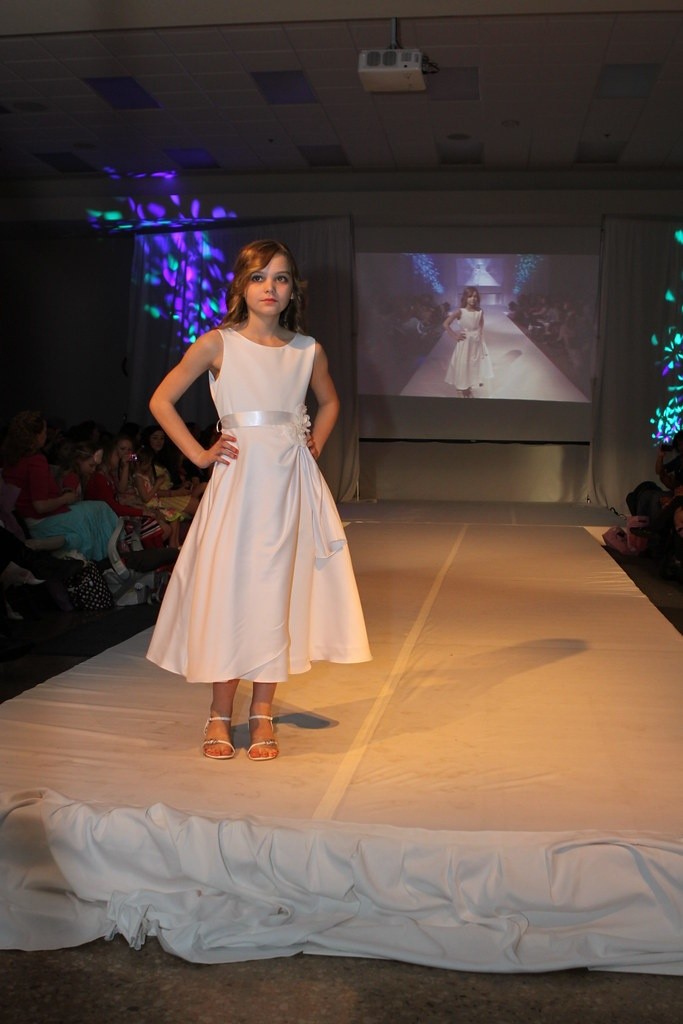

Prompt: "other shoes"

[626,522,670,543]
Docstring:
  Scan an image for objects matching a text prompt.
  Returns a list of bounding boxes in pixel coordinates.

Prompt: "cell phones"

[127,453,139,461]
[662,444,674,452]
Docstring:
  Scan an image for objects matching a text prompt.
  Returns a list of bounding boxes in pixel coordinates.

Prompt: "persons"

[597,429,683,590]
[1,404,214,635]
[351,251,596,404]
[146,239,371,758]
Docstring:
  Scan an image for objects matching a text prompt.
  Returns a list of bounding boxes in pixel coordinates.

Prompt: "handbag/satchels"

[603,516,652,556]
[65,563,114,622]
[100,569,148,606]
[625,481,668,522]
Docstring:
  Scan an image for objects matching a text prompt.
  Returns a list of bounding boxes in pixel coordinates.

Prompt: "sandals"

[247,715,280,762]
[203,716,236,759]
